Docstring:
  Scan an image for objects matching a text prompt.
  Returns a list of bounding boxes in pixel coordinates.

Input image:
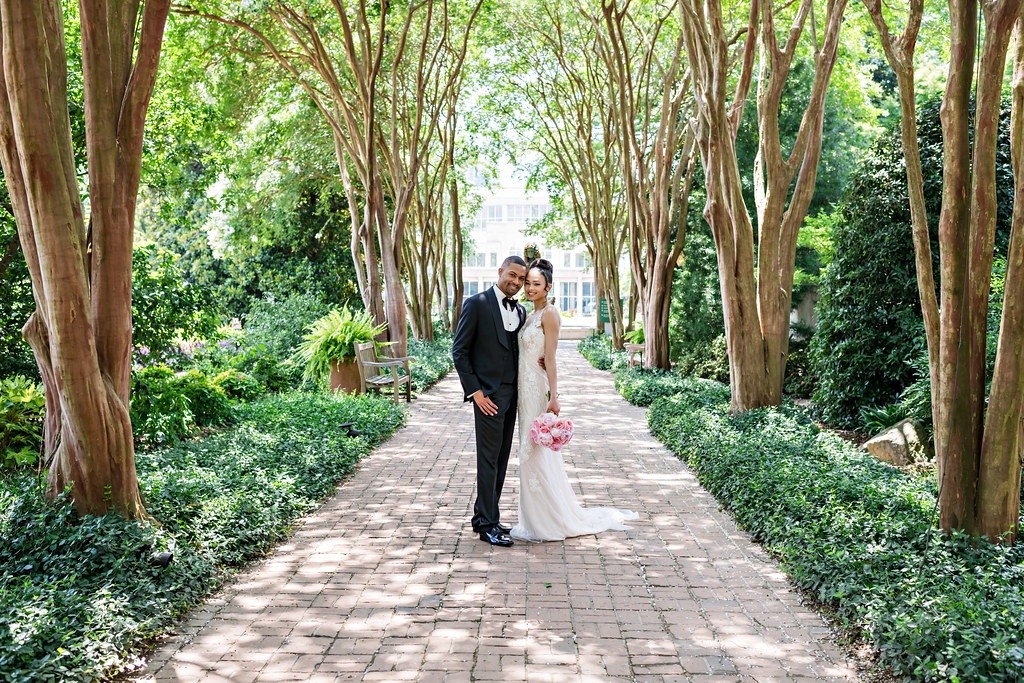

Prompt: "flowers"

[529,390,574,451]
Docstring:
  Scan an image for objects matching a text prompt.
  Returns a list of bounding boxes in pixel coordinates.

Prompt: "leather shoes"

[494,523,512,534]
[480,528,514,547]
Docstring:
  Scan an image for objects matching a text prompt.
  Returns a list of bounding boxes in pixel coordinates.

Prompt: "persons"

[510,257,636,541]
[453,256,547,547]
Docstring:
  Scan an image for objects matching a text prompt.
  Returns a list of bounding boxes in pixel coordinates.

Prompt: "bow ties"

[502,296,518,312]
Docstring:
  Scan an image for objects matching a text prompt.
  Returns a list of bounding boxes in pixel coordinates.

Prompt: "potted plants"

[291,299,400,396]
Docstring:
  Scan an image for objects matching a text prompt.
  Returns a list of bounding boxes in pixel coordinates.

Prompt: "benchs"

[353,340,416,403]
[623,343,645,367]
